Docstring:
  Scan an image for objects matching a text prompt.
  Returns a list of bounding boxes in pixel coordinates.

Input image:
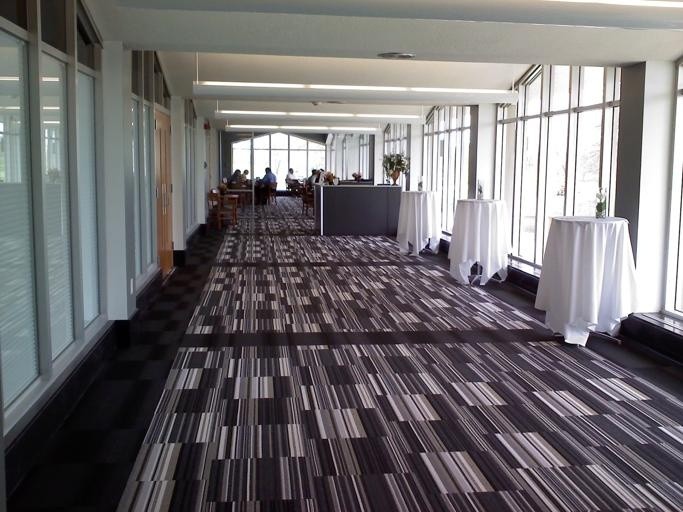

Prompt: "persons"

[241,169,248,181]
[284,166,325,198]
[230,169,241,182]
[254,167,276,206]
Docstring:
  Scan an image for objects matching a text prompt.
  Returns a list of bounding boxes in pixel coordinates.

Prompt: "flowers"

[381,152,410,185]
[596,188,607,218]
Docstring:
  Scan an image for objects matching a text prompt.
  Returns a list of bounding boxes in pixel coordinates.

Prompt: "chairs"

[286,179,314,216]
[206,178,277,231]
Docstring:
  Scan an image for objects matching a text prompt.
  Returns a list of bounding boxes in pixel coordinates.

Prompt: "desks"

[448,199,514,286]
[535,217,639,347]
[396,191,443,257]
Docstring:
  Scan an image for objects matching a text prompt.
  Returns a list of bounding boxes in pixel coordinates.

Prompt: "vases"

[390,169,400,186]
[478,179,484,201]
[418,176,423,191]
[594,186,608,219]
